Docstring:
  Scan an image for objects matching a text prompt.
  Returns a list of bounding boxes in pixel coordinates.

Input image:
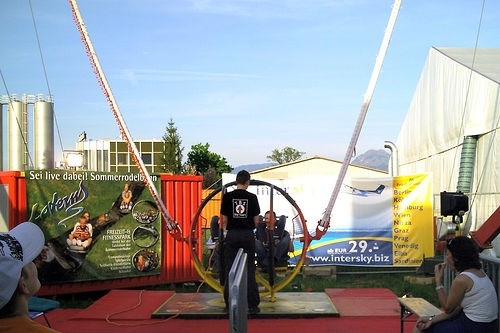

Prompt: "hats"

[0,222,46,309]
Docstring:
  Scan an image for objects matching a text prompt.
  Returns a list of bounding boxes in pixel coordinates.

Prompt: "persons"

[207,215,227,273]
[414,237,498,333]
[0,221,60,333]
[254,211,294,273]
[66,210,93,250]
[219,170,261,316]
[120,182,134,215]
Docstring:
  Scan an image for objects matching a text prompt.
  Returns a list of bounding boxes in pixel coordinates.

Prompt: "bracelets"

[435,284,443,292]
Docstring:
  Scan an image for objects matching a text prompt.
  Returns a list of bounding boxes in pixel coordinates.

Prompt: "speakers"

[440,191,469,217]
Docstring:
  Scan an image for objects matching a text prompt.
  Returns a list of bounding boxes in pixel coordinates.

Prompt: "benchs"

[398,298,441,333]
